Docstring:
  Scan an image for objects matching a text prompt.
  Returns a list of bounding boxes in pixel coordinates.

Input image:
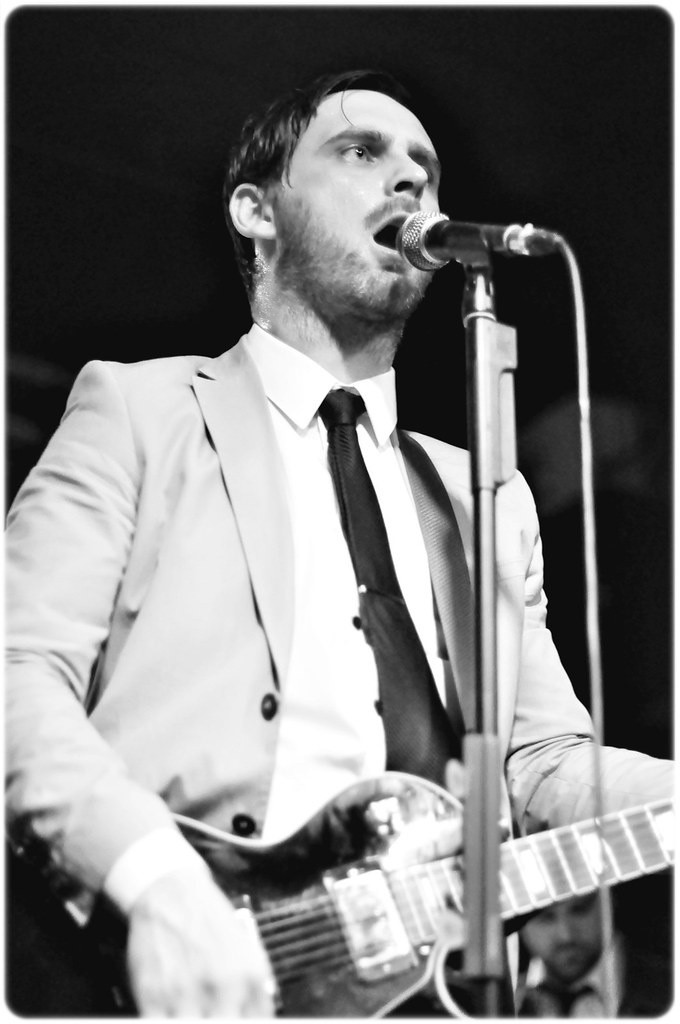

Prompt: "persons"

[5,68,673,1018]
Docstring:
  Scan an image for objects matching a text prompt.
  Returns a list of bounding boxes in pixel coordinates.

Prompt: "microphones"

[396,211,561,271]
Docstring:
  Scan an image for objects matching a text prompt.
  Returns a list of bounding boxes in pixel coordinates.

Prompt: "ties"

[316,386,464,789]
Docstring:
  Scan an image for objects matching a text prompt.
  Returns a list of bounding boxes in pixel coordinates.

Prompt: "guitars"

[4,770,675,1019]
